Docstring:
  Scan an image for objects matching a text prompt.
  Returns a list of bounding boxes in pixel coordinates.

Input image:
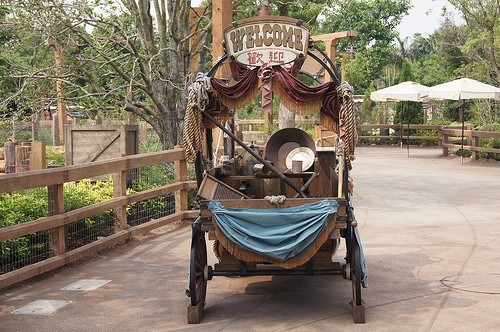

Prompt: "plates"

[286,147,315,171]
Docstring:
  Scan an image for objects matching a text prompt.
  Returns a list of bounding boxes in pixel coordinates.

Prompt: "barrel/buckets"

[264,127,316,173]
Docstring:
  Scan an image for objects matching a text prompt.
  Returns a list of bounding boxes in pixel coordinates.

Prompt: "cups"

[254,164,270,175]
[292,160,302,174]
